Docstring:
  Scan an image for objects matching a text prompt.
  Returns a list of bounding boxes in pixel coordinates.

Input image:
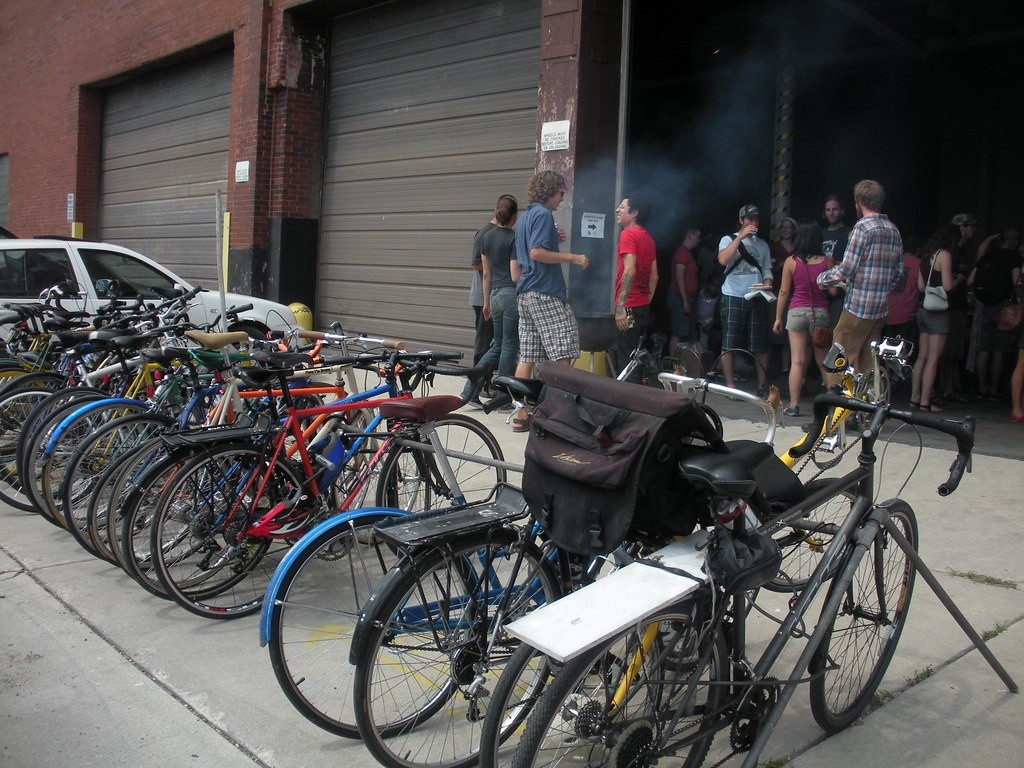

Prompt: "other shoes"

[716,370,747,383]
[756,389,769,400]
[726,394,746,402]
[789,383,808,396]
[779,368,789,378]
[941,390,1006,403]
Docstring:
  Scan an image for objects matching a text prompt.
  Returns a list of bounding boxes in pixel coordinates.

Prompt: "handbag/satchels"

[996,301,1024,332]
[811,326,833,348]
[923,285,948,311]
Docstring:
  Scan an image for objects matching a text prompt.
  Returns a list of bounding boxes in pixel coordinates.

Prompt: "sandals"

[909,401,944,413]
[512,413,532,432]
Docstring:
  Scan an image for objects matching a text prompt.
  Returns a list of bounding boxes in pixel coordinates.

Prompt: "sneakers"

[461,396,484,410]
[801,422,814,433]
[1008,413,1024,424]
[783,403,800,417]
[478,383,498,398]
[845,413,859,430]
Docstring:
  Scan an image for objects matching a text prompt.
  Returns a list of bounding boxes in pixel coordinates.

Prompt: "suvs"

[0,232,302,390]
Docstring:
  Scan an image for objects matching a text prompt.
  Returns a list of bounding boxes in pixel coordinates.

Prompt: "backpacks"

[972,247,1012,305]
[519,360,731,558]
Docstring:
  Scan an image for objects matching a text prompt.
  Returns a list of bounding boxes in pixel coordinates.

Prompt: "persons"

[511,170,590,433]
[610,193,660,385]
[664,179,1024,424]
[459,194,528,414]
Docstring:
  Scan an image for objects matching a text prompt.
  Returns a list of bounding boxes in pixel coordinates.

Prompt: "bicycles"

[2,277,989,768]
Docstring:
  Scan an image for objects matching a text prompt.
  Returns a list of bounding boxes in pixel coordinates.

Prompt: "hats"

[780,217,799,233]
[951,212,980,227]
[739,204,761,218]
